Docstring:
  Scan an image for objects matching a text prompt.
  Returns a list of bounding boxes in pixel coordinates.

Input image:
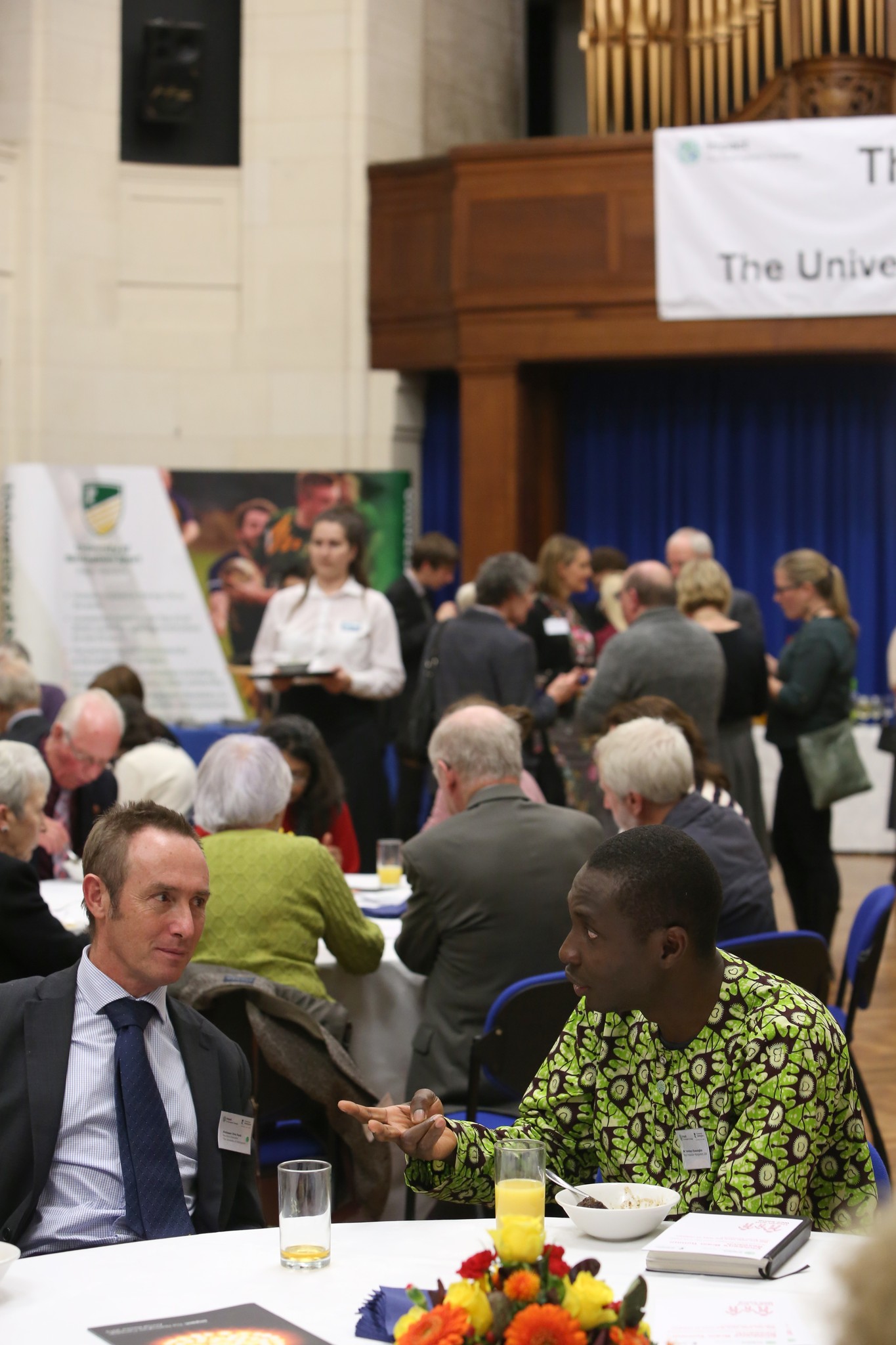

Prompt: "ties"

[106,997,197,1240]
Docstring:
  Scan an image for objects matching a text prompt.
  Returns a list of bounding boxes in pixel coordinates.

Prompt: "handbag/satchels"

[798,717,874,810]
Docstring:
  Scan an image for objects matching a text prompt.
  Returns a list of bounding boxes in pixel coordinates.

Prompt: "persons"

[0,737,93,983]
[337,828,878,1237]
[0,800,277,1258]
[177,732,384,1049]
[394,704,605,1111]
[5,473,895,946]
[594,714,776,946]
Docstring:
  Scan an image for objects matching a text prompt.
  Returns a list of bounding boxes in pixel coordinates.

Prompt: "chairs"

[165,720,896,1205]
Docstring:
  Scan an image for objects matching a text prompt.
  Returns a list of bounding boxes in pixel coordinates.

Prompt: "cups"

[376,839,404,885]
[494,1139,547,1232]
[277,1158,332,1270]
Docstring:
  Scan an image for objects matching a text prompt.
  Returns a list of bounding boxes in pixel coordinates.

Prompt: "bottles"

[857,695,870,722]
[870,693,883,722]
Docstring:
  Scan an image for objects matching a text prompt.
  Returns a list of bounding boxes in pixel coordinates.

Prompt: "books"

[644,1211,813,1279]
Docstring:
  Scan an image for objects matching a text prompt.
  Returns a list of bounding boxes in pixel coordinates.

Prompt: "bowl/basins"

[0,1240,21,1285]
[271,662,311,672]
[555,1181,681,1241]
[61,858,84,882]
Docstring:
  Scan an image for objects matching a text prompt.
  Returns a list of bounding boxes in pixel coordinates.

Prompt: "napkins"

[356,1285,446,1343]
[358,898,409,918]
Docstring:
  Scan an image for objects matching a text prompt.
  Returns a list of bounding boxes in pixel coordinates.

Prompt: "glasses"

[64,729,114,771]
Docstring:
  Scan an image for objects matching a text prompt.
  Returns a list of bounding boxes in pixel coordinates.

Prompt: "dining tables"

[36,869,441,1217]
[0,1218,865,1345]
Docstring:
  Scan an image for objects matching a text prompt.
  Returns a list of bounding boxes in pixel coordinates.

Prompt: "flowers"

[392,1214,655,1345]
